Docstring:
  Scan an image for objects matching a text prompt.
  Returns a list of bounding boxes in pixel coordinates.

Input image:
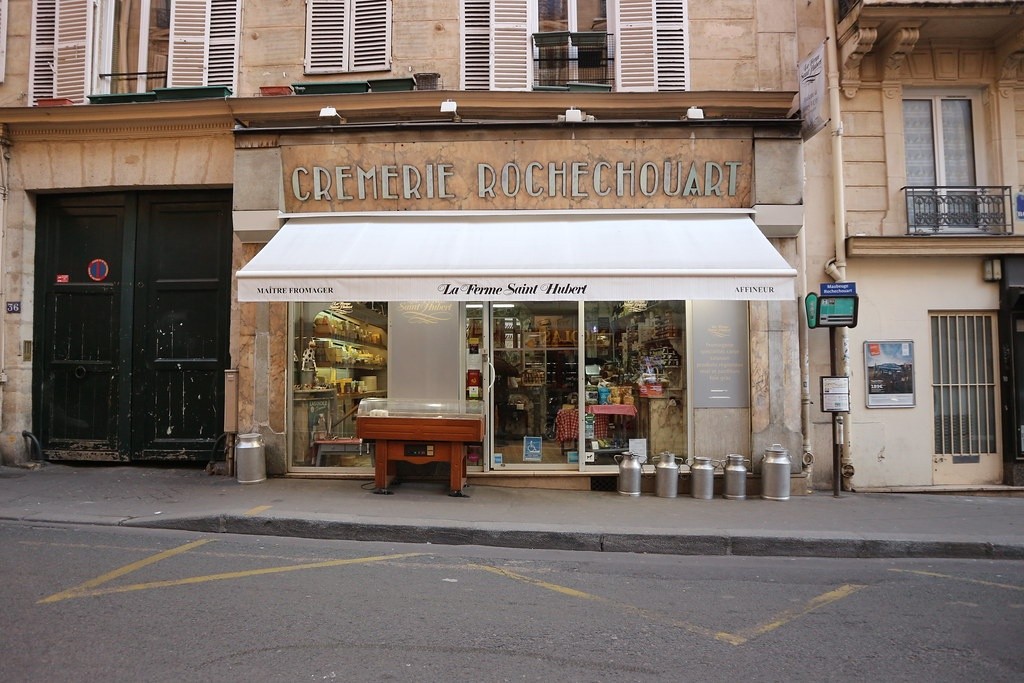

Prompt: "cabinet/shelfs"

[308,308,387,426]
[610,301,687,392]
[311,438,376,468]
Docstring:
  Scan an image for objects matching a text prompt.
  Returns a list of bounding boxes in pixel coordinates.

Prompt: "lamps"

[318,106,348,124]
[679,105,705,122]
[566,106,583,124]
[438,99,462,123]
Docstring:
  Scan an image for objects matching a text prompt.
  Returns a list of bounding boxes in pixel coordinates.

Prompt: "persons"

[493,346,520,448]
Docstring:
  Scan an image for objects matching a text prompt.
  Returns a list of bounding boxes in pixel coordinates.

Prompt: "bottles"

[652,450,684,498]
[586,384,635,407]
[234,433,269,484]
[758,442,793,503]
[720,452,751,500]
[687,455,720,500]
[613,451,648,497]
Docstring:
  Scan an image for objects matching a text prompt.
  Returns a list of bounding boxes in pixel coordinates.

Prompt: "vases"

[413,72,444,91]
[86,92,155,103]
[36,97,76,107]
[291,79,371,94]
[567,81,612,93]
[151,85,233,99]
[531,85,570,93]
[259,85,294,96]
[365,77,417,91]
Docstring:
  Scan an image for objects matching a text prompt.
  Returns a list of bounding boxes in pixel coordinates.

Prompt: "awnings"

[234,207,799,303]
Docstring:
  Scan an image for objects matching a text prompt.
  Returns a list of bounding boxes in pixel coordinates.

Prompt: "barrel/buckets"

[639,379,650,396]
[298,368,318,388]
[646,379,664,397]
